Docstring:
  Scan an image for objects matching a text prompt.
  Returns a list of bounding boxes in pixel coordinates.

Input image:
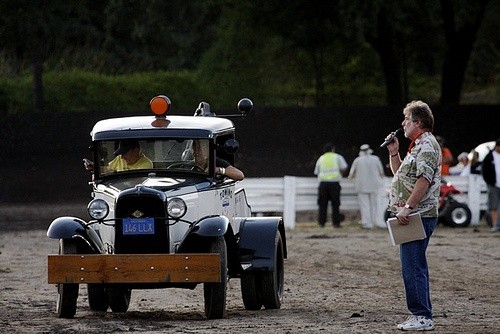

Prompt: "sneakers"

[396,315,435,331]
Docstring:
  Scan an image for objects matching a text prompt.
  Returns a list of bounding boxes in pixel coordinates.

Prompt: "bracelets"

[390,153,398,157]
[219,167,225,174]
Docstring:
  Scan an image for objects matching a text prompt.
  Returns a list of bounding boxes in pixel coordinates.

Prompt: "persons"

[480,140,500,233]
[385,101,442,331]
[439,142,451,175]
[313,143,347,228]
[348,145,388,229]
[447,152,469,173]
[83,141,152,174]
[182,139,244,182]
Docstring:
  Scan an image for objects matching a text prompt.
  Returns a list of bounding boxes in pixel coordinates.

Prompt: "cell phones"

[85,160,92,167]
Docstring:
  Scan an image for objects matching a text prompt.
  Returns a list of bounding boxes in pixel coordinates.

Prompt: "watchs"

[405,204,414,210]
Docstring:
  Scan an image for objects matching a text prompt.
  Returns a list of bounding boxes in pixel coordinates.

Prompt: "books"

[387,214,426,246]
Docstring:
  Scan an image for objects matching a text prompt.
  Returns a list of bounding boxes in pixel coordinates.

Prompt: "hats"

[359,144,373,156]
[113,138,143,155]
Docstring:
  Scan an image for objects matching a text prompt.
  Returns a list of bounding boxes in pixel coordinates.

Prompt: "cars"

[439,141,500,228]
[47,95,288,321]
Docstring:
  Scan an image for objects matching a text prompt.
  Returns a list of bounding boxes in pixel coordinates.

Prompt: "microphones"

[380,128,404,147]
